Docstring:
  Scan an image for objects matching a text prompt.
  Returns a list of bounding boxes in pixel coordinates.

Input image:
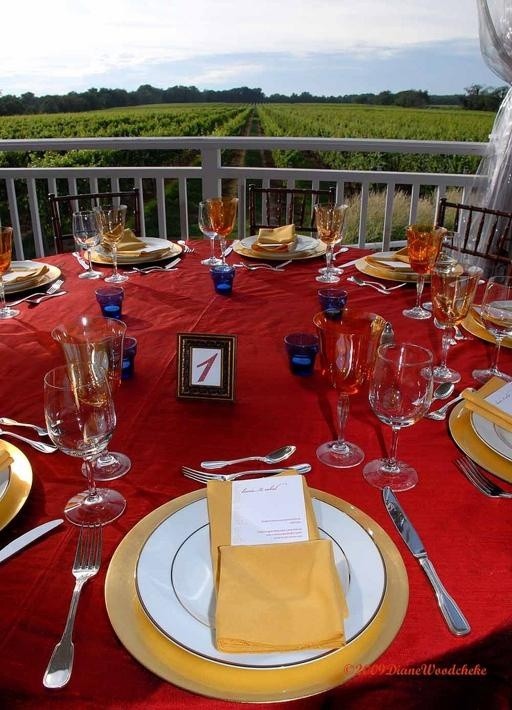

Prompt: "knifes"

[381,484,470,635]
[220,239,240,258]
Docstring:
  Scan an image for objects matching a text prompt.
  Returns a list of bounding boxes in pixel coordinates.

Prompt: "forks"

[122,256,182,272]
[181,463,312,484]
[42,522,102,687]
[347,277,408,289]
[1,418,50,436]
[176,240,194,253]
[455,457,511,500]
[232,256,292,269]
[452,324,468,342]
[427,388,473,421]
[7,279,63,305]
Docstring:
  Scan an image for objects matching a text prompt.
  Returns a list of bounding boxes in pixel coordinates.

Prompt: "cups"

[103,334,136,380]
[285,333,317,376]
[316,289,348,322]
[209,264,235,294]
[94,286,123,322]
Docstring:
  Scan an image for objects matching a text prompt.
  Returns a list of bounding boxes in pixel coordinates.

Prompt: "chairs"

[48,188,141,253]
[248,184,336,236]
[436,197,512,286]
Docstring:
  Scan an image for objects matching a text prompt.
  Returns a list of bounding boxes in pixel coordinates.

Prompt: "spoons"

[201,446,294,469]
[431,382,456,404]
[323,246,349,260]
[241,261,286,273]
[24,292,68,306]
[352,276,391,296]
[0,430,58,455]
[134,266,180,275]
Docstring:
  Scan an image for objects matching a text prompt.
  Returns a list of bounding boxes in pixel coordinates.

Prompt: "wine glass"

[43,364,126,526]
[206,198,240,272]
[472,275,512,388]
[317,322,365,469]
[52,316,132,480]
[197,201,223,266]
[0,227,20,320]
[72,210,104,279]
[420,264,483,384]
[97,203,129,284]
[314,204,346,284]
[401,224,445,319]
[363,342,435,490]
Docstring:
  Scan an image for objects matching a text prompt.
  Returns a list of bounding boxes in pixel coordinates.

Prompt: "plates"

[1,439,32,532]
[355,251,463,283]
[447,380,511,486]
[84,236,183,268]
[0,261,44,295]
[247,233,319,256]
[105,478,411,704]
[138,489,385,671]
[470,381,512,464]
[1,261,61,296]
[94,236,171,259]
[460,305,511,350]
[234,233,327,261]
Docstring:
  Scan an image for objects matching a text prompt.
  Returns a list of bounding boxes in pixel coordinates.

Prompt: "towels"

[368,249,452,276]
[207,471,320,588]
[463,377,512,435]
[104,227,143,250]
[254,222,296,255]
[472,301,512,331]
[395,244,439,267]
[0,267,51,289]
[215,539,348,653]
[102,229,168,261]
